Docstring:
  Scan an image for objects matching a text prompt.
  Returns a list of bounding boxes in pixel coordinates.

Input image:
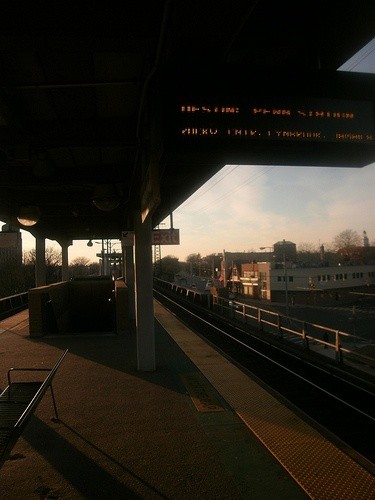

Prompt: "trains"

[152,276,214,313]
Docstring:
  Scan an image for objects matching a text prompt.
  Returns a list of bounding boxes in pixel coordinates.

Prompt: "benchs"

[0,347,69,476]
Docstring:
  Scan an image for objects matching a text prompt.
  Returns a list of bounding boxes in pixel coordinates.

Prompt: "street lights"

[260,246,289,316]
